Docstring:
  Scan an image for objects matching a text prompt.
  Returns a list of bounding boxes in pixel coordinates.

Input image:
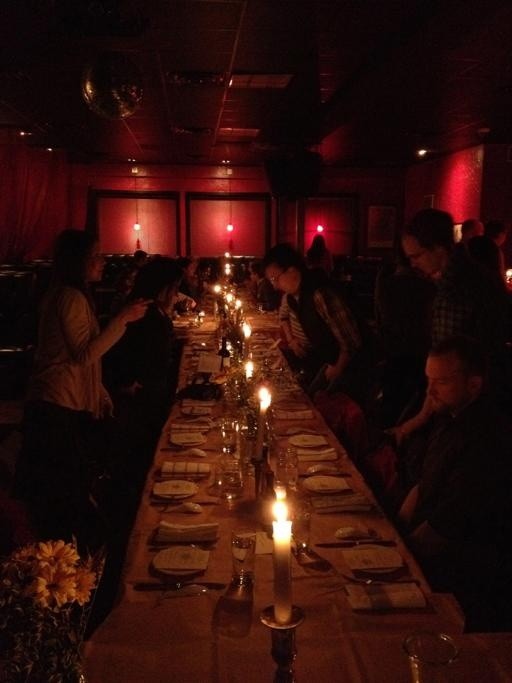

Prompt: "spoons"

[332,524,371,541]
[306,462,338,473]
[159,502,203,514]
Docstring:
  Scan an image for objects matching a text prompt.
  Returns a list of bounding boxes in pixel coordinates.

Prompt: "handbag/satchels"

[178,375,222,400]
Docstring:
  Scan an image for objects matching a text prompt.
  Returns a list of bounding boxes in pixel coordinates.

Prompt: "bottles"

[252,445,275,506]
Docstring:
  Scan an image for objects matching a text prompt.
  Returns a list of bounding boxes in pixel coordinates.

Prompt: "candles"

[264,479,302,622]
[243,360,255,385]
[257,383,278,413]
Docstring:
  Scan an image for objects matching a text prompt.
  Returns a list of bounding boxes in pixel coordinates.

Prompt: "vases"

[27,607,88,681]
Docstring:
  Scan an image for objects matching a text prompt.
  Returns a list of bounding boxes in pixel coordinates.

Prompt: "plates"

[302,476,343,494]
[153,546,203,575]
[155,480,197,499]
[161,333,325,476]
[346,541,404,575]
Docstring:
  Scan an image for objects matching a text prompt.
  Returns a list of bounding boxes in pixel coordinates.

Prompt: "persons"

[23,228,213,549]
[230,210,511,629]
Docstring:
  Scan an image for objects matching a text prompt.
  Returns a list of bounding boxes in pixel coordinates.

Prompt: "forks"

[342,573,418,585]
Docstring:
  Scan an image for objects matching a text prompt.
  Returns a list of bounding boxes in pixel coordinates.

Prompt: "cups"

[213,575,254,636]
[221,472,241,512]
[292,545,330,571]
[400,628,457,682]
[230,525,257,584]
[275,446,300,494]
[289,497,313,555]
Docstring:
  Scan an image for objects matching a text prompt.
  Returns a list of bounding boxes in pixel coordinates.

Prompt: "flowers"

[2,537,99,650]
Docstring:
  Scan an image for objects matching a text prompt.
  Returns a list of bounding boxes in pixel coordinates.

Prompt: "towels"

[344,582,429,612]
[275,410,373,512]
[100,305,468,680]
[153,393,226,548]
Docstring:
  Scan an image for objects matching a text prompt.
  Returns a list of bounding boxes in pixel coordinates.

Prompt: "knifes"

[133,581,227,592]
[314,538,398,550]
[150,499,220,505]
